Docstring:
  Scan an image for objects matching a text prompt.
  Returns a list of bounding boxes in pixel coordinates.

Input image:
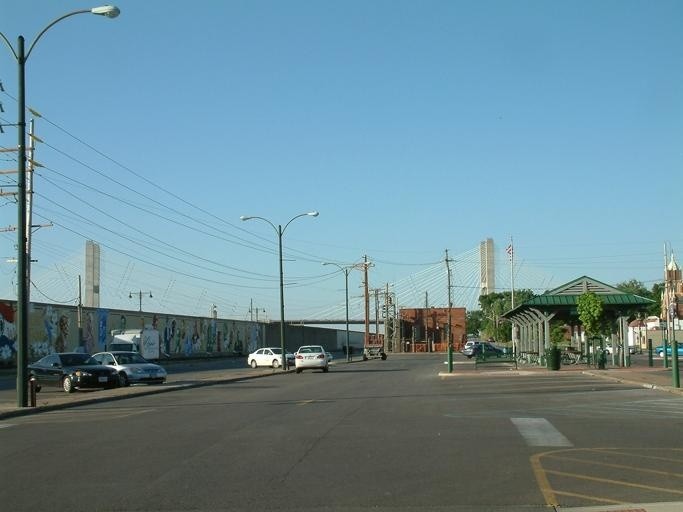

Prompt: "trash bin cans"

[544,348,561,370]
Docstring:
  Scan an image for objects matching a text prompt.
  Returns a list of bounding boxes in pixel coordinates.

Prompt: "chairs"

[474,352,517,370]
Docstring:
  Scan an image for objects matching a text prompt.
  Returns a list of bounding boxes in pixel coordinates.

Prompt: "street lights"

[0,5,119,407]
[320,261,370,361]
[238,211,318,370]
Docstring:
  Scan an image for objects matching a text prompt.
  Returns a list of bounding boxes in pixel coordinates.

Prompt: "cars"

[655,341,682,358]
[565,342,637,360]
[26,352,120,393]
[247,343,332,373]
[84,350,166,387]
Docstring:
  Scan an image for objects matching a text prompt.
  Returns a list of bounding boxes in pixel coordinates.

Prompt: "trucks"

[106,327,160,361]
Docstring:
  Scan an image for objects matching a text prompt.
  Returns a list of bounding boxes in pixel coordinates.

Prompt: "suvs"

[361,344,387,360]
[462,341,496,358]
